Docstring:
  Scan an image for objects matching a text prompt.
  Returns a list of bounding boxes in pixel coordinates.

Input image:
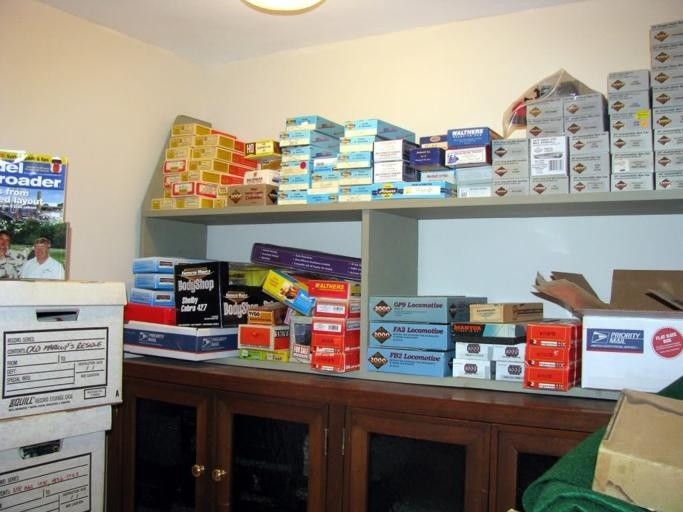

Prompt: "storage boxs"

[160,115,280,208]
[494,94,611,194]
[591,387,683,512]
[1,281,130,512]
[371,268,682,400]
[136,243,363,374]
[280,116,497,204]
[609,19,683,194]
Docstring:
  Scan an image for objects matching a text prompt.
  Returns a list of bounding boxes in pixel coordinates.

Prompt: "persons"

[0,230,19,279]
[19,238,65,279]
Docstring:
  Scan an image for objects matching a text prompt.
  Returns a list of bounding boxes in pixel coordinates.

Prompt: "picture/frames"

[0,150,70,280]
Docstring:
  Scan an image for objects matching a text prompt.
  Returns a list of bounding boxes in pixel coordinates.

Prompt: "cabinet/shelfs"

[138,115,683,406]
[112,355,615,512]
